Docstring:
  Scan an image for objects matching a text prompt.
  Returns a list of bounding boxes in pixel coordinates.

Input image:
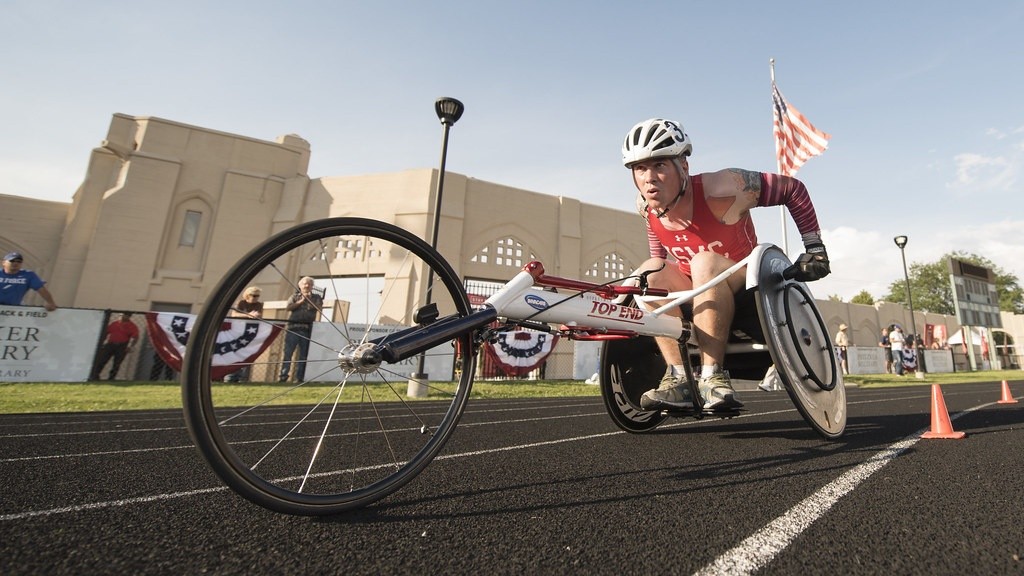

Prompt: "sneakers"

[695,370,744,412]
[640,363,699,414]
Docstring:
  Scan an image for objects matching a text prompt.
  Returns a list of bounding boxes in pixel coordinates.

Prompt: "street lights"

[411,97,464,379]
[894,236,920,372]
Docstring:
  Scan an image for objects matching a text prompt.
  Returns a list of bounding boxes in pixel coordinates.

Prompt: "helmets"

[623,117,692,166]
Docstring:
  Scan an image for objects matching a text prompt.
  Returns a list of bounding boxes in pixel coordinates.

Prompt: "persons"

[879,324,951,376]
[835,324,856,375]
[147,351,173,381]
[220,286,263,383]
[277,276,323,383]
[88,311,140,381]
[619,118,830,412]
[758,364,786,391]
[0,252,55,311]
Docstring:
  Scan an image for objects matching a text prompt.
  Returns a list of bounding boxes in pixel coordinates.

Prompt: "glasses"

[249,293,260,297]
[10,259,22,264]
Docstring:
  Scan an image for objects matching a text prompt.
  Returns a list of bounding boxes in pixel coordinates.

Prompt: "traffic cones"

[997,380,1019,403]
[919,383,965,439]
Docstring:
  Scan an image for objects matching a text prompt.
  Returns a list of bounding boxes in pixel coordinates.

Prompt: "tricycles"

[180,216,847,515]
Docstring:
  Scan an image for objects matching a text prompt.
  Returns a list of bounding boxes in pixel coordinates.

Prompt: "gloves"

[783,243,829,282]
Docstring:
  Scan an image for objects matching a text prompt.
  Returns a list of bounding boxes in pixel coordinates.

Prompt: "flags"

[771,82,831,177]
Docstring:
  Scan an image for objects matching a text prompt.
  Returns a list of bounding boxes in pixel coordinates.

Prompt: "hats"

[839,323,848,331]
[895,324,902,329]
[2,253,24,263]
